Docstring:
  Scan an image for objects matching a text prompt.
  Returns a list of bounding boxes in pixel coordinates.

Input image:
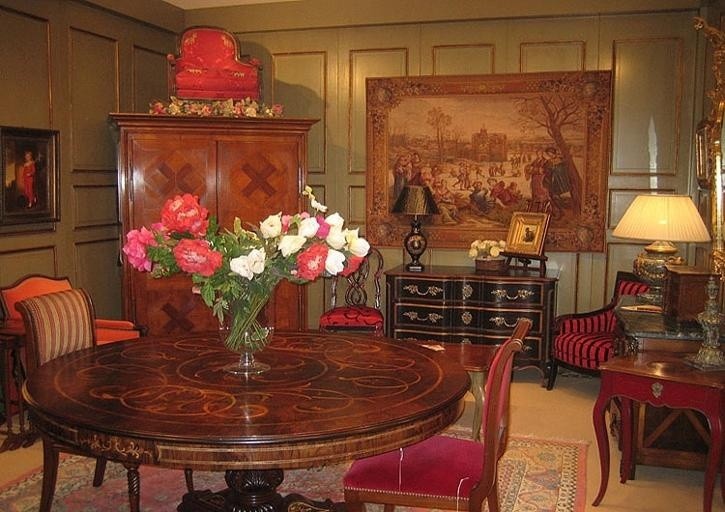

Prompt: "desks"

[591,348,725,512]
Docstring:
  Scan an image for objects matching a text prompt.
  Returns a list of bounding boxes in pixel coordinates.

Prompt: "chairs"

[319,248,383,336]
[344,318,534,511]
[13,286,194,511]
[0,272,148,450]
[546,271,651,391]
[166,26,263,102]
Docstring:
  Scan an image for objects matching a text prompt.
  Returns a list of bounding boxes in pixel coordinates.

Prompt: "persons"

[394,143,565,222]
[23,152,38,209]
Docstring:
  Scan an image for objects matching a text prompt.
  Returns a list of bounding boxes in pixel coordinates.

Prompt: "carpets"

[0,424,589,511]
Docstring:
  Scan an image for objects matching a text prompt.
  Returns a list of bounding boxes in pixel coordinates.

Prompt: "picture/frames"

[0,126,61,225]
[505,211,552,256]
[366,70,614,252]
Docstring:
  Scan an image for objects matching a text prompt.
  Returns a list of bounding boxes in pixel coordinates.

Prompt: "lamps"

[612,193,713,305]
[392,183,441,273]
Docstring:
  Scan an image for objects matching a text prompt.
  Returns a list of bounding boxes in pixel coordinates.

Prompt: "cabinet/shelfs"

[106,113,322,336]
[384,263,562,388]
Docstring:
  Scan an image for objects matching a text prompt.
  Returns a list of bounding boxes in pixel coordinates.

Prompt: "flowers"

[468,239,506,260]
[122,186,371,349]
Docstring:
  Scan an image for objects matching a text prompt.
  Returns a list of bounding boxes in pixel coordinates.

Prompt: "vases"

[213,286,275,374]
[475,259,507,274]
